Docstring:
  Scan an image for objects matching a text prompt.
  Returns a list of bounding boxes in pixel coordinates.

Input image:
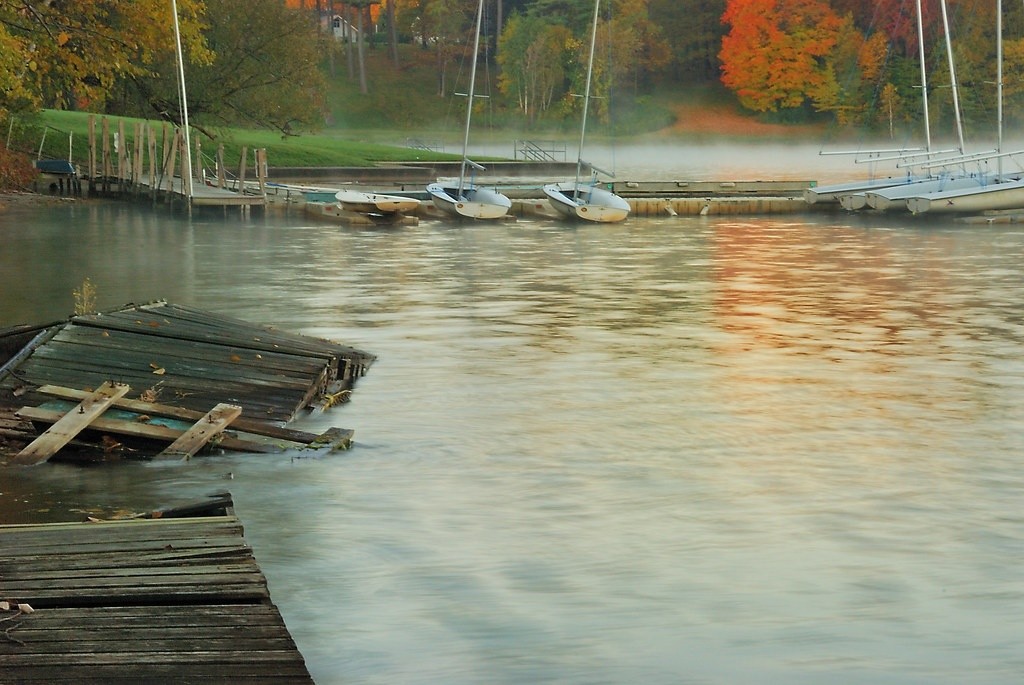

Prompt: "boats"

[335,188,421,225]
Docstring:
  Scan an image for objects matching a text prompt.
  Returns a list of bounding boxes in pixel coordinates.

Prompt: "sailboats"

[541,0,631,223]
[804,0,1024,214]
[424,0,512,220]
